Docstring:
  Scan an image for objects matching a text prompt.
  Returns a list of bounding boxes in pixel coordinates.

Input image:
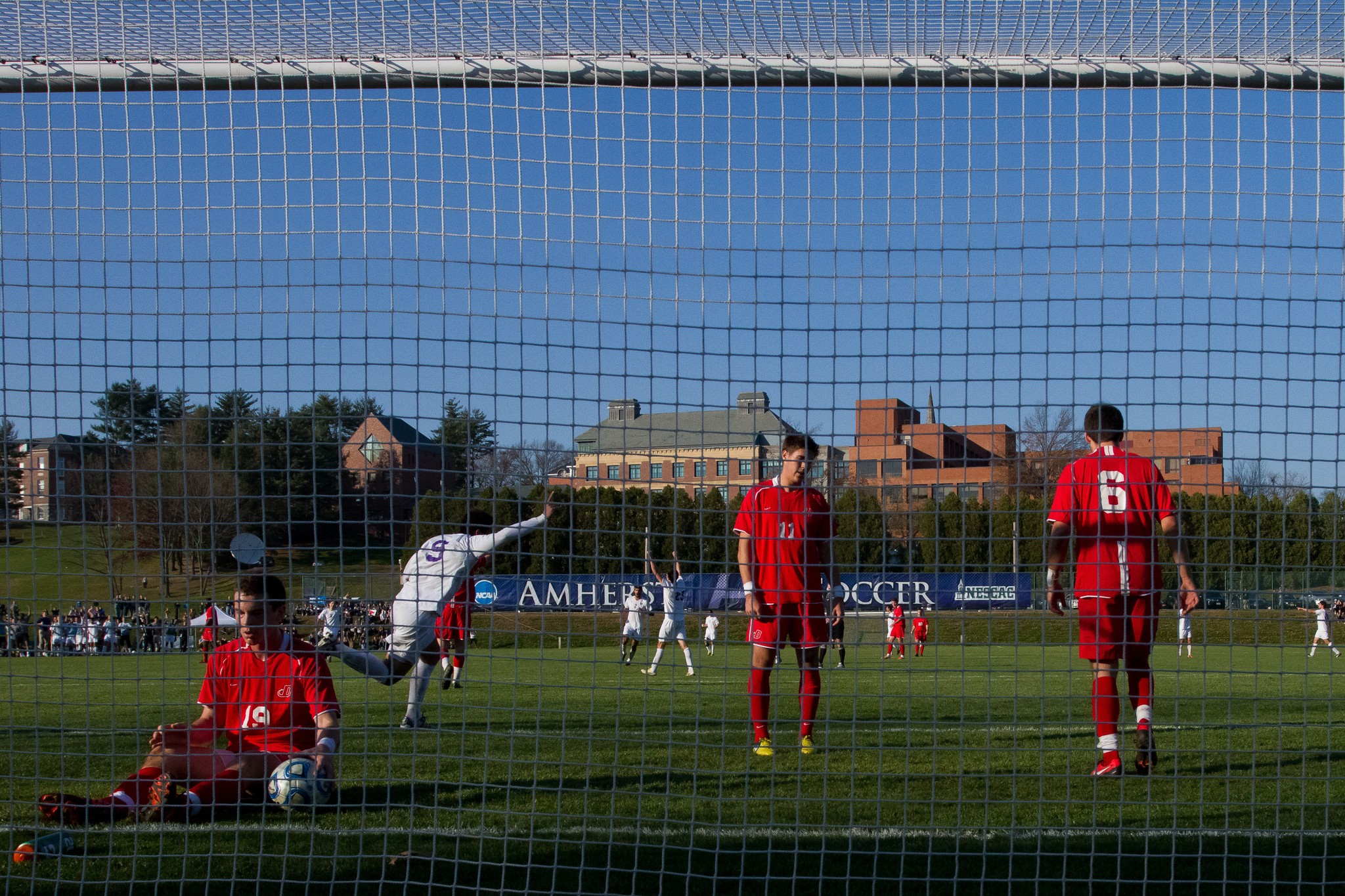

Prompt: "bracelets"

[833,585,843,598]
[317,738,336,753]
[1047,568,1061,587]
[742,581,756,595]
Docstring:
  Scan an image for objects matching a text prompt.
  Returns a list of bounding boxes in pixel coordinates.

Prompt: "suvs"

[1329,590,1345,603]
[1298,590,1332,609]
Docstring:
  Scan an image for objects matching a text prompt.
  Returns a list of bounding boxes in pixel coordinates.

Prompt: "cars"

[1269,594,1299,609]
[1194,592,1226,610]
[312,561,326,566]
[1237,594,1268,609]
[1059,594,1079,609]
[257,556,275,567]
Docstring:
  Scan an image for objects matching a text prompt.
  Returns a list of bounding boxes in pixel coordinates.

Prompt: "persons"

[37,573,339,826]
[1178,609,1194,658]
[1296,601,1341,657]
[435,554,492,690]
[1225,589,1230,610]
[280,592,392,663]
[1331,599,1345,624]
[1047,402,1199,776]
[733,433,845,757]
[819,583,846,668]
[314,490,560,728]
[621,551,719,675]
[142,577,147,588]
[883,598,929,660]
[0,594,239,664]
[1242,589,1250,609]
[1273,587,1282,610]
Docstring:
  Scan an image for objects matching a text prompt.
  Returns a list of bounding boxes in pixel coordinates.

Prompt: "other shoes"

[707,651,711,655]
[775,658,782,664]
[327,656,332,663]
[711,653,714,656]
[915,654,924,657]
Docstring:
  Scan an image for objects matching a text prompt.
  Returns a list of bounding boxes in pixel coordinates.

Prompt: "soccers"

[268,757,331,813]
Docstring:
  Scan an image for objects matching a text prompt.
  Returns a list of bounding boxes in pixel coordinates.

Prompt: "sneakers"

[686,672,696,676]
[453,682,463,688]
[1090,757,1121,777]
[641,668,657,676]
[818,663,823,669]
[442,664,454,689]
[753,737,774,755]
[1307,654,1314,658]
[1335,651,1342,657]
[401,714,438,730]
[622,651,627,661]
[801,736,817,754]
[897,656,906,660]
[314,632,339,654]
[1134,729,1157,773]
[145,772,178,823]
[881,655,891,660]
[39,794,114,825]
[897,648,900,655]
[835,662,845,668]
[1179,654,1195,658]
[626,661,630,666]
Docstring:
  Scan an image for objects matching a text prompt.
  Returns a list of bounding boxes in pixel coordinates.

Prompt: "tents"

[190,605,237,651]
